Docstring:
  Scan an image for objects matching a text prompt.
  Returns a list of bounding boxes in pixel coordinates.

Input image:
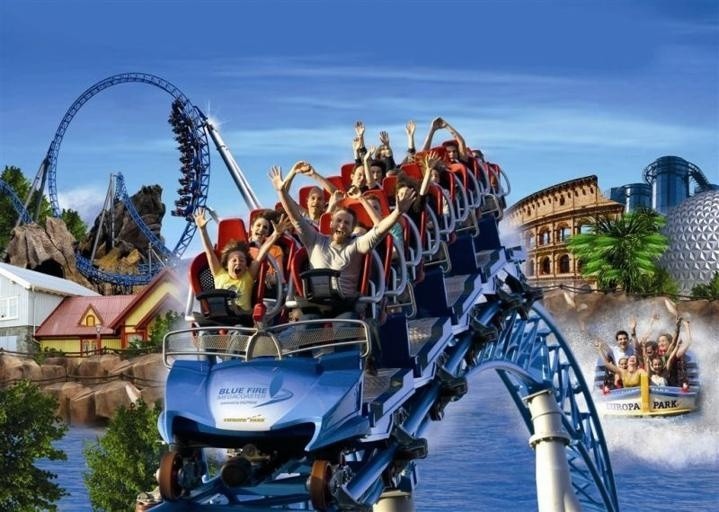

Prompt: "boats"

[592,340,701,417]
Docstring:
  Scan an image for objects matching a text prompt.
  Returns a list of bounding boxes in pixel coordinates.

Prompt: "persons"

[590,311,692,388]
[183,116,469,316]
